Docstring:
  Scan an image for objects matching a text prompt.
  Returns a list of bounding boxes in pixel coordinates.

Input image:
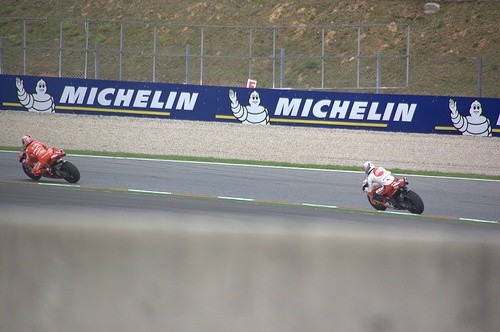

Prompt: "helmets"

[363,161,375,174]
[21,135,32,146]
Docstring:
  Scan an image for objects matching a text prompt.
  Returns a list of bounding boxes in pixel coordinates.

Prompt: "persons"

[361,160,407,207]
[20,134,64,176]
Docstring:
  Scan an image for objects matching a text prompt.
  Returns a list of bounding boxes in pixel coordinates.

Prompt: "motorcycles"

[362,176,425,215]
[18,146,81,183]
